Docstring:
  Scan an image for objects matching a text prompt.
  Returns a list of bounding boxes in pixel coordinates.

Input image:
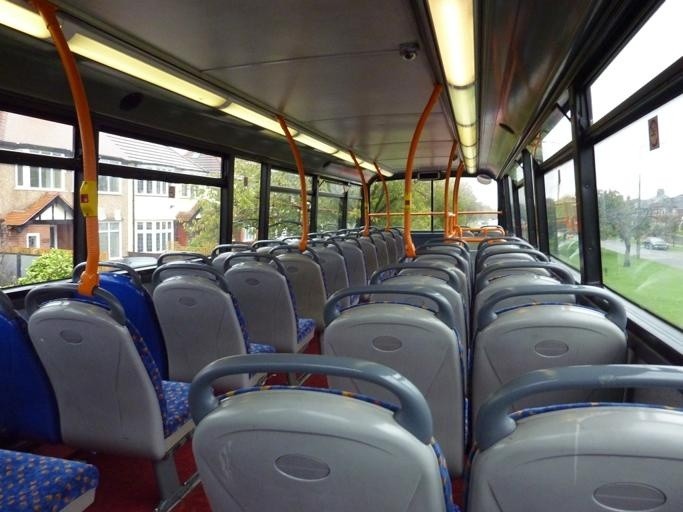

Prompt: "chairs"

[3,293,60,446]
[70,262,167,372]
[323,286,463,473]
[223,252,315,349]
[357,223,586,327]
[156,227,368,304]
[3,451,96,510]
[278,254,328,334]
[471,282,627,416]
[464,364,680,510]
[151,262,275,389]
[26,282,193,500]
[189,352,455,510]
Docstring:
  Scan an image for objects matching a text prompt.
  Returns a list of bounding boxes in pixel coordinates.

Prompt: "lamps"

[0,0,485,177]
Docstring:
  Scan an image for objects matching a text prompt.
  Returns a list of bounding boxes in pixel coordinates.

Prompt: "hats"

[643,237,668,249]
